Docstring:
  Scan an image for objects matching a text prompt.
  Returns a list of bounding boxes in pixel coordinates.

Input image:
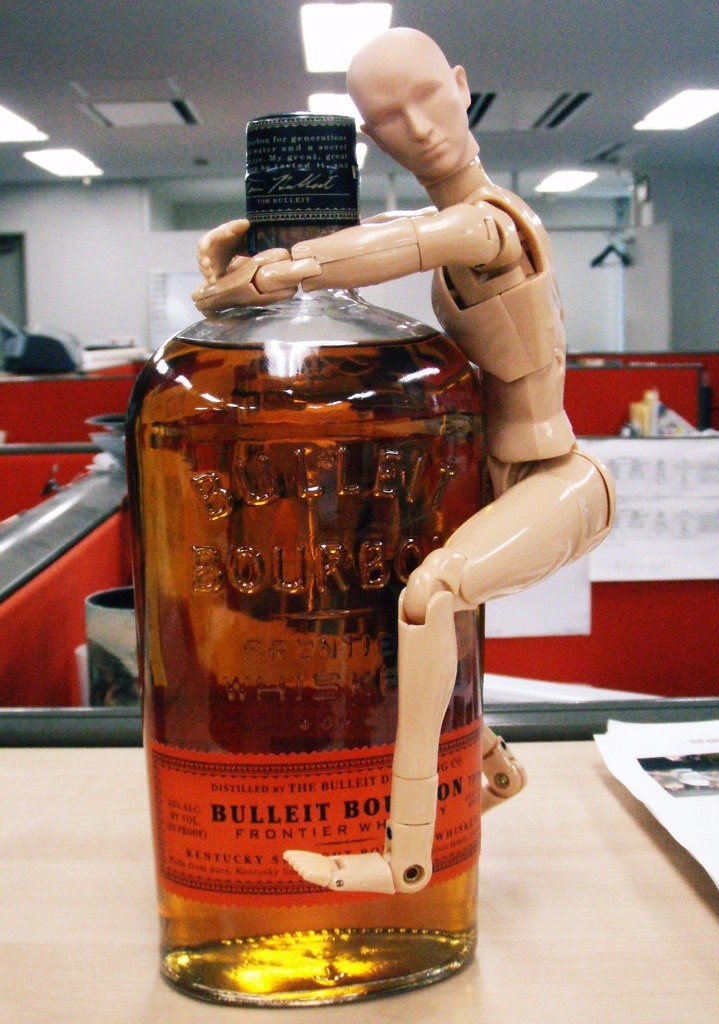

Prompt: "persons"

[192,27,616,895]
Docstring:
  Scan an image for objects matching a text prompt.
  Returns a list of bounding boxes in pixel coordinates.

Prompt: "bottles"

[124,113,483,1010]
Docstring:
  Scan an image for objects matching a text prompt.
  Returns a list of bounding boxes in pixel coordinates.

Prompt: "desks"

[1,741,719,1024]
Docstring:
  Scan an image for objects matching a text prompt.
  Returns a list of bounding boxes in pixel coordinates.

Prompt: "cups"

[83,585,143,707]
